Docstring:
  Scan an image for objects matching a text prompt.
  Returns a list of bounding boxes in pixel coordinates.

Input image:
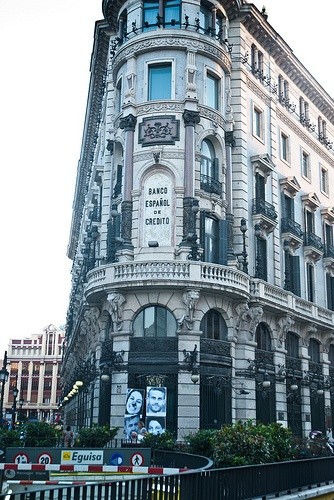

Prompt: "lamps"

[148,241,159,247]
[178,345,203,384]
[276,361,299,393]
[60,356,97,406]
[236,352,271,395]
[300,370,334,395]
[99,344,138,383]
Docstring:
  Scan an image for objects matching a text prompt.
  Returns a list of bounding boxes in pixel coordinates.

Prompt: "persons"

[125,389,144,414]
[147,417,165,435]
[148,388,165,412]
[64,426,73,447]
[125,415,140,440]
[3,421,9,429]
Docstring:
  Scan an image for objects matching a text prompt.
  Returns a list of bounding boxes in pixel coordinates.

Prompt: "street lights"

[19,397,24,429]
[12,386,19,430]
[0,359,9,428]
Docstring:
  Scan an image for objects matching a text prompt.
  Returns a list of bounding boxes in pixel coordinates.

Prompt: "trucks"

[6,412,17,425]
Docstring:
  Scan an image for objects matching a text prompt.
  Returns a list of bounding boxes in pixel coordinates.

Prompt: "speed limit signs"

[37,453,52,464]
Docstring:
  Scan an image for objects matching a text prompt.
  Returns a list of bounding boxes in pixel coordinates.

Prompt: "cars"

[15,420,24,430]
